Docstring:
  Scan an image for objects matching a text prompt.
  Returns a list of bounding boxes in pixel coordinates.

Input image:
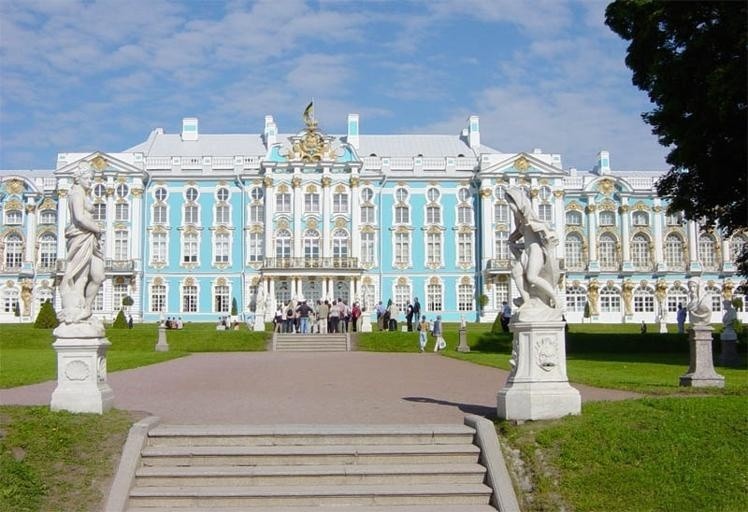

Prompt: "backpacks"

[287,309,292,316]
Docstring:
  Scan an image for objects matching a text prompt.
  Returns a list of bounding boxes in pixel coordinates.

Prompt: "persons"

[217,315,239,329]
[273,297,361,334]
[688,277,712,326]
[504,186,562,314]
[676,304,686,336]
[166,317,183,330]
[128,315,134,329]
[59,163,107,308]
[500,300,512,333]
[723,300,736,329]
[373,296,443,352]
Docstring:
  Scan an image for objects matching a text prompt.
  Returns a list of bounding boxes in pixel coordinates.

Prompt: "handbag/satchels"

[417,325,421,331]
[438,341,446,349]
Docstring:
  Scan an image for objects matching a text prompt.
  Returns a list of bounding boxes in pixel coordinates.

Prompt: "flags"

[304,102,314,124]
